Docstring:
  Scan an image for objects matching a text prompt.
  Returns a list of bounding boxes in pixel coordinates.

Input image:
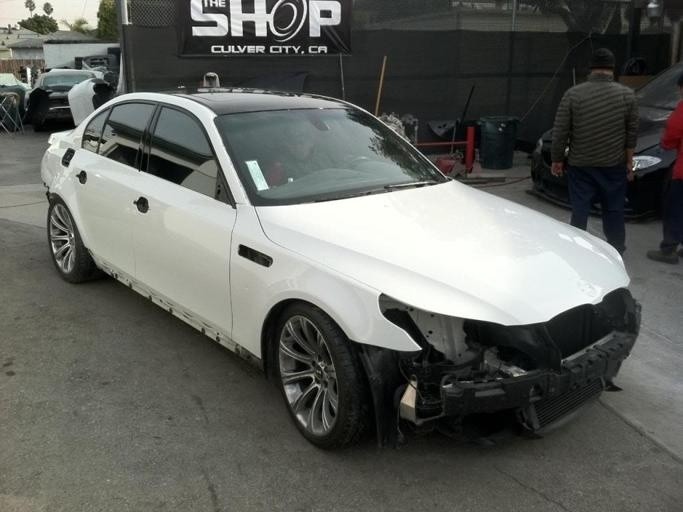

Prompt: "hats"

[588,48,617,69]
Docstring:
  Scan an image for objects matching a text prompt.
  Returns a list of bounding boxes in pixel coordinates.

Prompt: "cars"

[0,66,115,132]
[531,62,683,219]
[41,87,641,451]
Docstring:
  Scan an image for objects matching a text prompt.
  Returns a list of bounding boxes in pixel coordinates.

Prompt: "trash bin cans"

[477,116,514,169]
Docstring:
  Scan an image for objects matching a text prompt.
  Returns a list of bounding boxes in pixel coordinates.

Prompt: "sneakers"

[646,247,683,262]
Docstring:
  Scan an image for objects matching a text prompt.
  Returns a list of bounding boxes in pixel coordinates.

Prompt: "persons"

[646,74,683,264]
[549,44,639,254]
